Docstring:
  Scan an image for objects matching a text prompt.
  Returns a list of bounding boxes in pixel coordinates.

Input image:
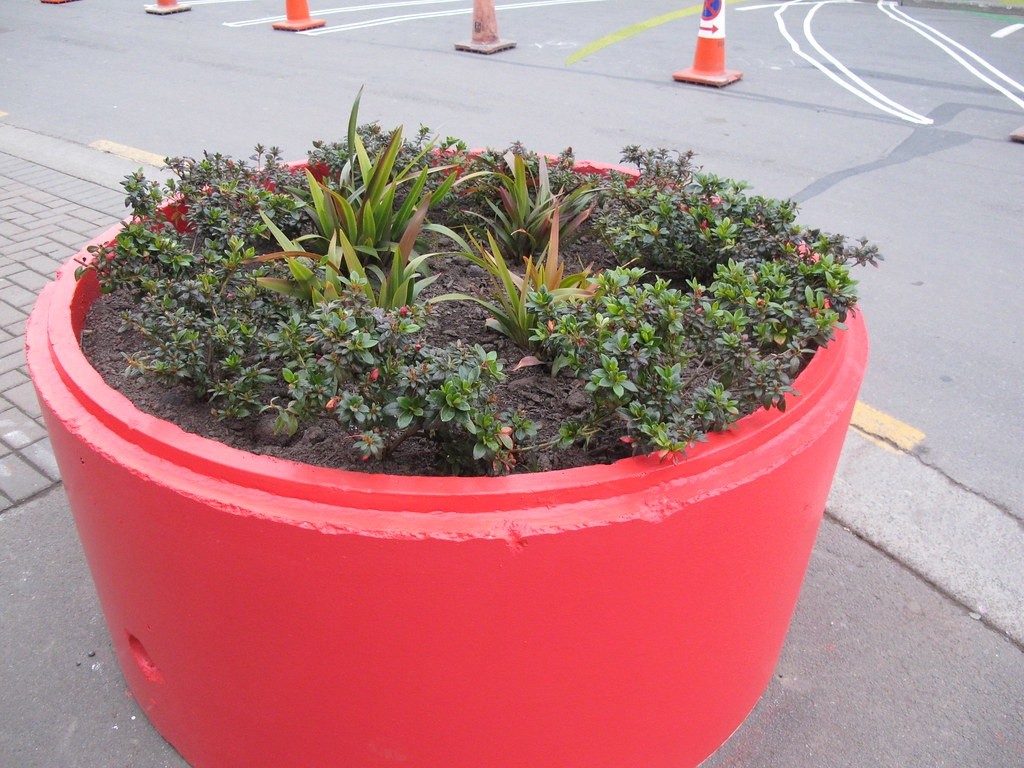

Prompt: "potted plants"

[25,147,868,768]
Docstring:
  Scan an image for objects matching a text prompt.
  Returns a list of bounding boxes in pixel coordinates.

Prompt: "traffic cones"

[41,0,75,4]
[144,0,191,15]
[673,0,744,87]
[454,0,518,54]
[272,0,327,31]
